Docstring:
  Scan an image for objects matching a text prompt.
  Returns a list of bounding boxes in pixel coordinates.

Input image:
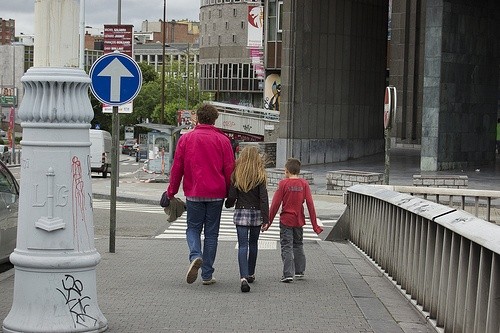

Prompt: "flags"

[8,106,16,148]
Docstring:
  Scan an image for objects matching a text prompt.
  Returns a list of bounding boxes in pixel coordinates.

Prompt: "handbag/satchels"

[160,191,169,207]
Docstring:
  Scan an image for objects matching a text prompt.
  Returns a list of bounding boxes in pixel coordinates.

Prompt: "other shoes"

[202,277,216,285]
[295,272,304,276]
[240,278,251,292]
[186,257,202,284]
[280,276,293,282]
[248,275,255,283]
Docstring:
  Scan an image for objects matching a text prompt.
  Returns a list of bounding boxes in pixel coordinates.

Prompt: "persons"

[263,158,323,282]
[136,145,140,162]
[160,103,236,285]
[3,146,11,167]
[225,146,270,292]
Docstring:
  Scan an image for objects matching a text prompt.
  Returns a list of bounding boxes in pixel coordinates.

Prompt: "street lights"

[162,42,190,109]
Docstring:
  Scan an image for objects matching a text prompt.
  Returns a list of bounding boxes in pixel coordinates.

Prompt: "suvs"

[0,144,11,162]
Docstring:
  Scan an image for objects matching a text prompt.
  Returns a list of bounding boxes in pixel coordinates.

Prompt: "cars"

[136,144,149,162]
[0,128,6,137]
[121,140,137,154]
[0,160,20,264]
[128,142,139,156]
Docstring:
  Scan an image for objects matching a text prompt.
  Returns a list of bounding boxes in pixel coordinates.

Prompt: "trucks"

[88,129,112,178]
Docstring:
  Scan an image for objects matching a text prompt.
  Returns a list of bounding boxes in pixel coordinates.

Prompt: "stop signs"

[384,87,391,129]
